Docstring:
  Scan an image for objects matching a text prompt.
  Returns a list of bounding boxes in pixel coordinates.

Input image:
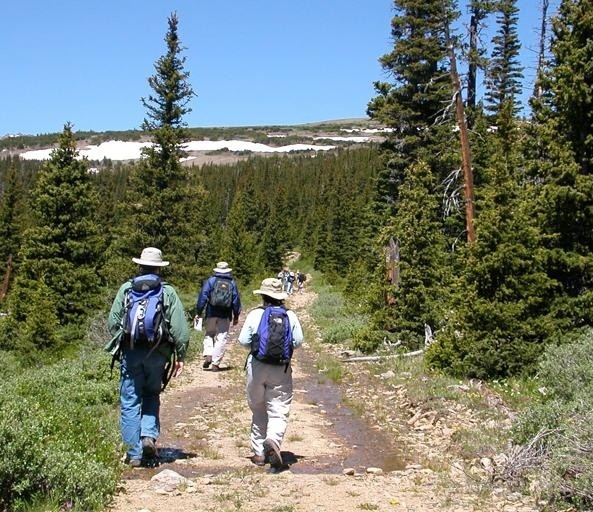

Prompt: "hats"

[130,246,169,269]
[212,261,233,275]
[251,277,289,301]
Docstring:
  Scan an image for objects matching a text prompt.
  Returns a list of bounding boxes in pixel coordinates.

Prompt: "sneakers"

[142,436,156,460]
[263,438,284,470]
[203,355,211,370]
[250,453,266,466]
[212,364,220,372]
[123,458,142,466]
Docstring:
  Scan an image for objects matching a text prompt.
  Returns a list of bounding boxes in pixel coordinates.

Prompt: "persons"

[194,261,242,372]
[238,276,304,468]
[104,247,190,469]
[278,269,306,295]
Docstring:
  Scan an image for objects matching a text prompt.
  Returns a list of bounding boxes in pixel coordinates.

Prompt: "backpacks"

[287,273,307,283]
[207,277,234,309]
[250,306,296,366]
[118,277,165,350]
